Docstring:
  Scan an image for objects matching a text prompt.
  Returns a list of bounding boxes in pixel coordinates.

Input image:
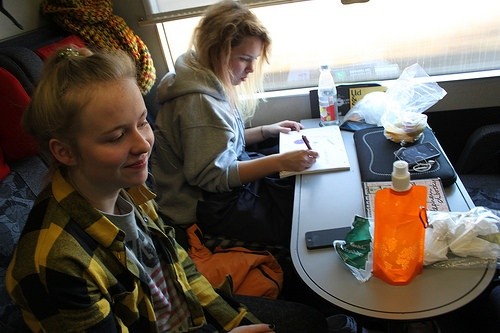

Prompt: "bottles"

[317,65,340,127]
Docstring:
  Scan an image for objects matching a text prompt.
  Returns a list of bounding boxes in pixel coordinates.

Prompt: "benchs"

[0,22,281,333]
[460,124,500,332]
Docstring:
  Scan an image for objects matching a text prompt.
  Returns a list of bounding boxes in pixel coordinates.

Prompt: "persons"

[144,0,319,251]
[0,44,365,333]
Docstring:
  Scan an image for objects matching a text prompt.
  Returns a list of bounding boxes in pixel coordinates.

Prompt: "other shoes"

[326,313,367,333]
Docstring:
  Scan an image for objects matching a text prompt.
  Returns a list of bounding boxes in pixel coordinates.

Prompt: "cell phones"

[393,142,441,167]
[305,227,351,250]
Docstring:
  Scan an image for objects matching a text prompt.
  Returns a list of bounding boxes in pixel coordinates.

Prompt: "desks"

[291,116,497,320]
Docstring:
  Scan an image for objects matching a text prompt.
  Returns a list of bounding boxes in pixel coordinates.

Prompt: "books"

[279,125,350,178]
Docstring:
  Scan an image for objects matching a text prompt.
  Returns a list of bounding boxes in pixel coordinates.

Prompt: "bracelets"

[261,126,266,141]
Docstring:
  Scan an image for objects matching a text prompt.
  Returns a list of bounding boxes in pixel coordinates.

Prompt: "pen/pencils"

[302,135,312,150]
